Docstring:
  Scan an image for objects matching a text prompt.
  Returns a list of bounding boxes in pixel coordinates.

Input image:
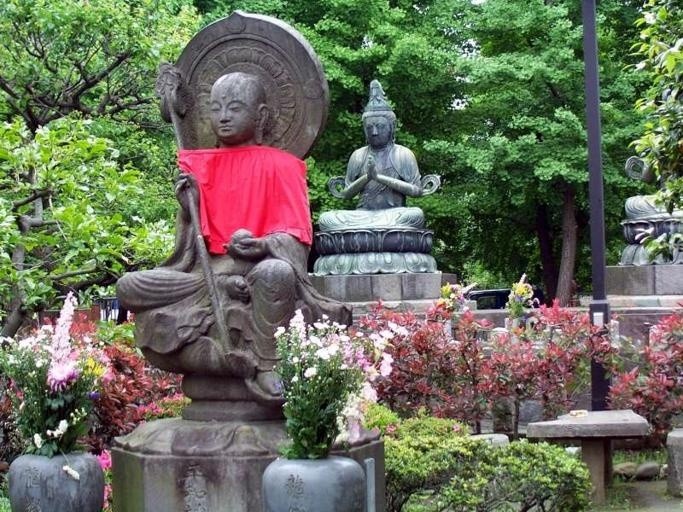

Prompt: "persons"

[117,72,313,395]
[319,79,425,230]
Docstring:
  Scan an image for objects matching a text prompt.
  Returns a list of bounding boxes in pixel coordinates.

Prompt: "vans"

[465,283,547,310]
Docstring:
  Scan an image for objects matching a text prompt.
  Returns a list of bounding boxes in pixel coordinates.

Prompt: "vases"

[504,317,525,330]
[8,452,105,512]
[260,459,368,512]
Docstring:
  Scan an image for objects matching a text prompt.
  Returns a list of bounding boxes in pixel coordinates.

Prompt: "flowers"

[0,292,106,479]
[262,307,407,457]
[437,281,479,317]
[504,273,540,321]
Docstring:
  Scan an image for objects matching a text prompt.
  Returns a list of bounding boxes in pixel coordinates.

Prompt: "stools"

[666,429,683,497]
[469,432,510,446]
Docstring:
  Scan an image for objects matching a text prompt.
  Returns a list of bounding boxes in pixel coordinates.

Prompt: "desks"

[526,409,649,505]
